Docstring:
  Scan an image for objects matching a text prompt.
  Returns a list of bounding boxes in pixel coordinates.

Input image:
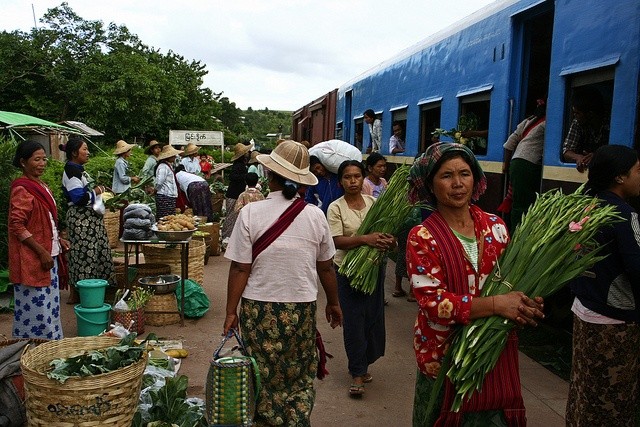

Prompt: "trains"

[290,0,639,219]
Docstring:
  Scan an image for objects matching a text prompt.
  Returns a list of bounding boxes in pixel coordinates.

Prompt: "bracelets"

[491,293,496,316]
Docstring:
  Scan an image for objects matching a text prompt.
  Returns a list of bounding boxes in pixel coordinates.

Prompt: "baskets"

[206,191,224,212]
[114,261,125,288]
[128,263,170,287]
[141,238,207,288]
[20,335,150,427]
[102,208,121,248]
[194,223,220,256]
[110,290,146,334]
[143,292,181,326]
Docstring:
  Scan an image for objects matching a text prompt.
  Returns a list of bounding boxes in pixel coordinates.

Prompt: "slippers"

[363,373,374,382]
[348,383,366,394]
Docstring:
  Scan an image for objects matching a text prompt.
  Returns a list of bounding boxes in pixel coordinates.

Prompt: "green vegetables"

[134,375,204,427]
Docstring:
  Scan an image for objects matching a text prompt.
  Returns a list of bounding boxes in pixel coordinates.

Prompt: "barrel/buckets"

[76,278,109,309]
[73,302,112,336]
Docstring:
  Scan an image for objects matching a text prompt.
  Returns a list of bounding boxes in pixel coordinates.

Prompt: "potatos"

[157,214,195,232]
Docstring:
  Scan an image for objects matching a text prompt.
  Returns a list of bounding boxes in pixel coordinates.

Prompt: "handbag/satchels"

[92,186,105,214]
[205,326,260,426]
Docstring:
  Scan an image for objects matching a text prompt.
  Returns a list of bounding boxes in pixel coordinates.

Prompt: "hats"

[184,143,201,157]
[155,144,184,162]
[143,140,164,155]
[255,139,318,186]
[113,140,135,154]
[230,143,252,162]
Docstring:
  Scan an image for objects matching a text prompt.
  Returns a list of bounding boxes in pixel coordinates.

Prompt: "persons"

[565,143,640,427]
[563,96,610,173]
[178,145,201,173]
[388,120,406,153]
[173,164,213,221]
[363,109,382,154]
[58,138,117,304]
[145,144,184,223]
[198,153,212,172]
[405,140,545,426]
[222,142,252,248]
[111,138,141,194]
[221,139,345,427]
[327,158,387,396]
[142,139,164,176]
[247,150,260,173]
[177,148,184,156]
[7,139,72,341]
[306,154,342,213]
[361,154,390,308]
[257,163,268,176]
[501,94,547,233]
[393,259,418,302]
[454,129,488,145]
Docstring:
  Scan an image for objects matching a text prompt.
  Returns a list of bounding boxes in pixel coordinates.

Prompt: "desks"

[119,233,191,326]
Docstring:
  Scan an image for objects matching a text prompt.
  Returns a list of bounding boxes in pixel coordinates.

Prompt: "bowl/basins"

[159,215,202,221]
[138,274,181,294]
[152,220,200,227]
[149,226,198,242]
